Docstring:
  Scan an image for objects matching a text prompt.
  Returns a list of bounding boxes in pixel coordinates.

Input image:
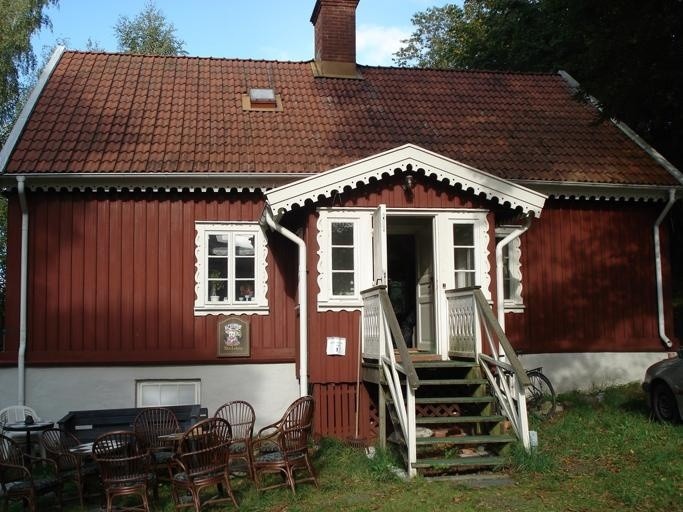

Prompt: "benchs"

[55,400,209,465]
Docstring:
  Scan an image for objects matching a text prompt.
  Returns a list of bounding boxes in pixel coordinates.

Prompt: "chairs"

[40,424,104,511]
[1,403,49,466]
[91,428,157,512]
[167,416,240,511]
[133,408,185,501]
[211,398,257,485]
[246,394,319,497]
[0,433,65,511]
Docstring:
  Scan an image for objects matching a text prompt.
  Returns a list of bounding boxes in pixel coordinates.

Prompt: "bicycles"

[478,352,556,423]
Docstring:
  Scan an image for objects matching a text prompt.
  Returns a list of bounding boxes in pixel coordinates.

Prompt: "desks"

[2,416,55,508]
[69,436,125,510]
[154,427,213,453]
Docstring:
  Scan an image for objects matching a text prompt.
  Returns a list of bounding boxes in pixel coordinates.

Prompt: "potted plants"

[206,267,226,302]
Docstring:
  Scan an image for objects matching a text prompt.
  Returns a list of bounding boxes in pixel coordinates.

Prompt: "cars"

[640,343,682,427]
